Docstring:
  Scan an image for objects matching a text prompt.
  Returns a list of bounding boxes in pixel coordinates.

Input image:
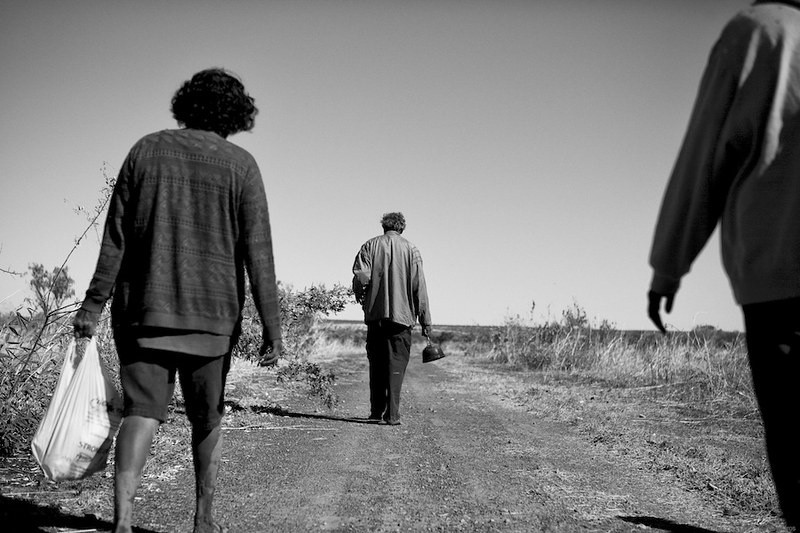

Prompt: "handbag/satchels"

[30,335,122,476]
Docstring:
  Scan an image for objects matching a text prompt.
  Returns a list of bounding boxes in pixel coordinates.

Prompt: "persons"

[646,0,800,533]
[351,211,432,426]
[70,67,283,533]
